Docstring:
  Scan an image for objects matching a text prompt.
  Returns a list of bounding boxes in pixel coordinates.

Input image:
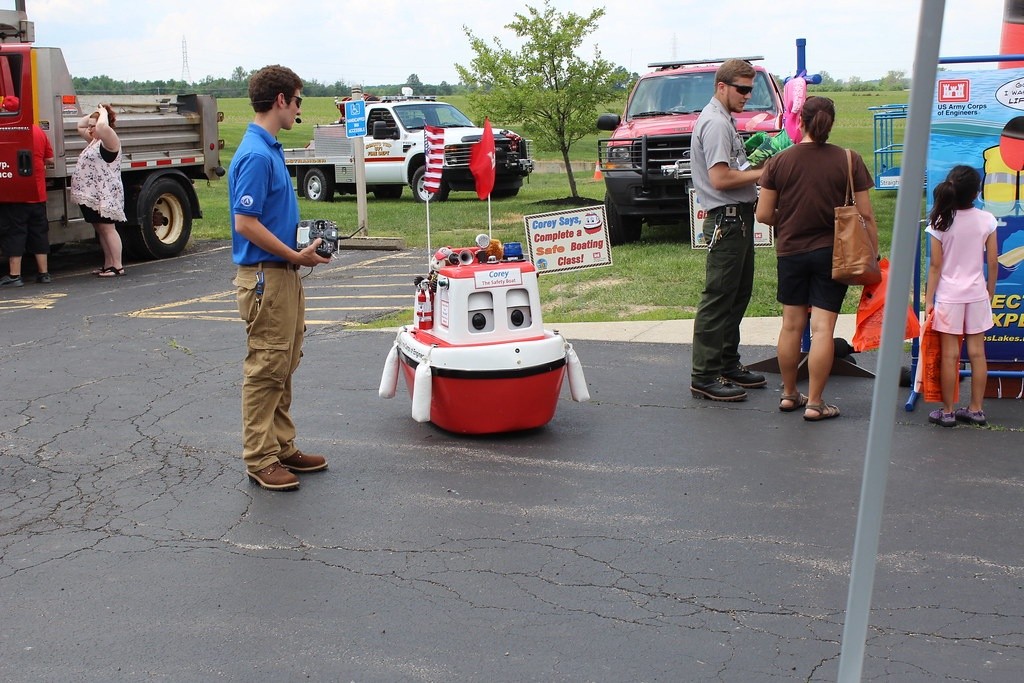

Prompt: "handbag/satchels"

[832,148,881,285]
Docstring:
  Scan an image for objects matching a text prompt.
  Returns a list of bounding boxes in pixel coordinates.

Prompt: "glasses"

[275,91,303,108]
[805,96,834,106]
[88,124,96,129]
[719,80,753,95]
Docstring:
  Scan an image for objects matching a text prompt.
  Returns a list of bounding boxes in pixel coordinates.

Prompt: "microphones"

[295,118,302,124]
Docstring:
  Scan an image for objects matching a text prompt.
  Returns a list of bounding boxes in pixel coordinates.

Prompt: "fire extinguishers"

[417,281,434,330]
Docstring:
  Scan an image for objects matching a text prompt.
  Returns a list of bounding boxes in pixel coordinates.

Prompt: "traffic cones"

[594,161,603,181]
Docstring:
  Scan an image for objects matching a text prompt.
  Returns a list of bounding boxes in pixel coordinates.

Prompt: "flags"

[471,120,495,199]
[423,126,445,193]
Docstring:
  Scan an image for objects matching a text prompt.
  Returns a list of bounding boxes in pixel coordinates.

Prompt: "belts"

[710,202,756,213]
[248,261,300,272]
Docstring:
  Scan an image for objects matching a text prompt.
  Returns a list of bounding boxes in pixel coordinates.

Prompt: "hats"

[0,96,20,112]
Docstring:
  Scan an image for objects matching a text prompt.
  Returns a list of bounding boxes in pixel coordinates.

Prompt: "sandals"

[778,390,809,411]
[91,265,107,275]
[803,398,840,420]
[99,267,126,278]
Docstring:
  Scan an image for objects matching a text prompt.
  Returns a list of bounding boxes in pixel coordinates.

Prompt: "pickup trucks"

[281,87,536,202]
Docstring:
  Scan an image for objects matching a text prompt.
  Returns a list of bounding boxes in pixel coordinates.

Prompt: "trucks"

[0,0,226,263]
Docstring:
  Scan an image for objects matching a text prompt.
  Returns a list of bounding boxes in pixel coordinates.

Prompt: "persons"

[926,165,998,427]
[0,97,54,286]
[690,59,766,402]
[757,96,875,421]
[71,105,127,277]
[230,68,331,490]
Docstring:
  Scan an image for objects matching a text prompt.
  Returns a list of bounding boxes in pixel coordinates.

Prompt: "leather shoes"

[690,375,747,402]
[281,450,328,472]
[719,361,767,387]
[246,461,300,491]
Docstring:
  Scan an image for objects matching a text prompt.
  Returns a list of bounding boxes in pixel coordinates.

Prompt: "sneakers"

[0,275,24,287]
[36,272,52,283]
[955,407,985,424]
[929,408,956,427]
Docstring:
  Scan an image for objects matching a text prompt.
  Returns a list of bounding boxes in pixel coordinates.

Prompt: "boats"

[378,113,590,437]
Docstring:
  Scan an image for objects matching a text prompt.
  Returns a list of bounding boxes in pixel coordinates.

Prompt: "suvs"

[594,56,789,244]
